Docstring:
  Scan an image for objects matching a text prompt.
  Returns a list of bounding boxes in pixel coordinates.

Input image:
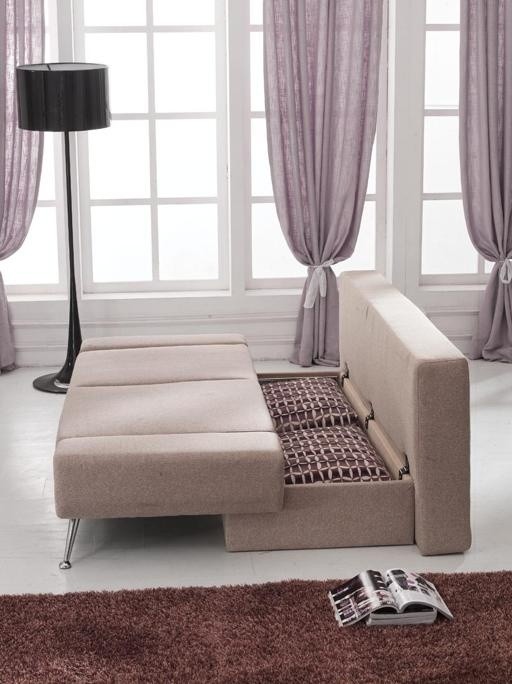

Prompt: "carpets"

[0,571,512,684]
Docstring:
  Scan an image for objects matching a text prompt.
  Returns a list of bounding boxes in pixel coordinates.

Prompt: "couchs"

[53,270,469,571]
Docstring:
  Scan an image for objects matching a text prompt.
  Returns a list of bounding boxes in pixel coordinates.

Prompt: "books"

[327,568,454,630]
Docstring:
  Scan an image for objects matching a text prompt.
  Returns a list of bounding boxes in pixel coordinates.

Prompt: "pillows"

[260,376,359,433]
[277,424,392,485]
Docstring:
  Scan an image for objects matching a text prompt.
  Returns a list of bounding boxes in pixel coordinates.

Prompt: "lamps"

[16,62,110,393]
